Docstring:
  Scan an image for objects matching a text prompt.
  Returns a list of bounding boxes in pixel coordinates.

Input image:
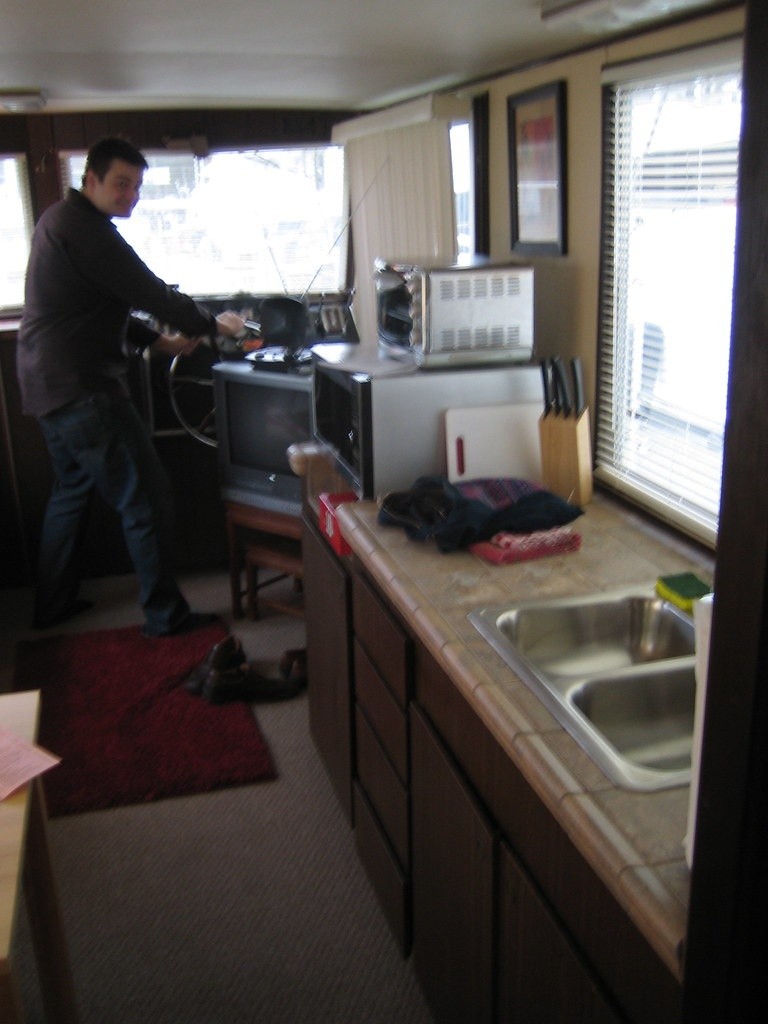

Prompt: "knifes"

[539,356,585,419]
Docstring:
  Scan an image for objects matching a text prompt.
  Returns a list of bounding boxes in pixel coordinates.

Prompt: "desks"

[226,503,302,622]
[0,689,74,1024]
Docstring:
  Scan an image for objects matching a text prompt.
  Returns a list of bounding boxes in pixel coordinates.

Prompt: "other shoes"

[158,611,224,639]
[25,592,95,627]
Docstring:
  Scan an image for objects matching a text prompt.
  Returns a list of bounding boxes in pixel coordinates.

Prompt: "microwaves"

[373,259,535,367]
[312,344,554,502]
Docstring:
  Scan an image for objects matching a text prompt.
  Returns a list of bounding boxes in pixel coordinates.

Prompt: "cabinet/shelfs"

[303,513,410,942]
[408,700,626,1024]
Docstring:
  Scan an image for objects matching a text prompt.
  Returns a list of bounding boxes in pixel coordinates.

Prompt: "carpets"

[7,611,280,818]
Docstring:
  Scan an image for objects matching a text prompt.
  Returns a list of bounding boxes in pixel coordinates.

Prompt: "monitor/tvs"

[212,359,316,520]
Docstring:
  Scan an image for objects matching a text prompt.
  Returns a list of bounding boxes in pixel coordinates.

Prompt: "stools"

[245,542,303,623]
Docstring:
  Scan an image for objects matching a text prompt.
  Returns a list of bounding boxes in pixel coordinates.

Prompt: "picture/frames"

[505,77,567,256]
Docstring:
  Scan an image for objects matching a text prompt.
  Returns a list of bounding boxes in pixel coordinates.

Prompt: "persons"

[17,137,245,638]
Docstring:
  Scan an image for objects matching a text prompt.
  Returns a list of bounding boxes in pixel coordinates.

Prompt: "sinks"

[494,591,699,673]
[563,667,699,791]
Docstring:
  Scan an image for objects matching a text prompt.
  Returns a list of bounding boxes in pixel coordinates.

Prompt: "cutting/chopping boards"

[445,403,553,485]
